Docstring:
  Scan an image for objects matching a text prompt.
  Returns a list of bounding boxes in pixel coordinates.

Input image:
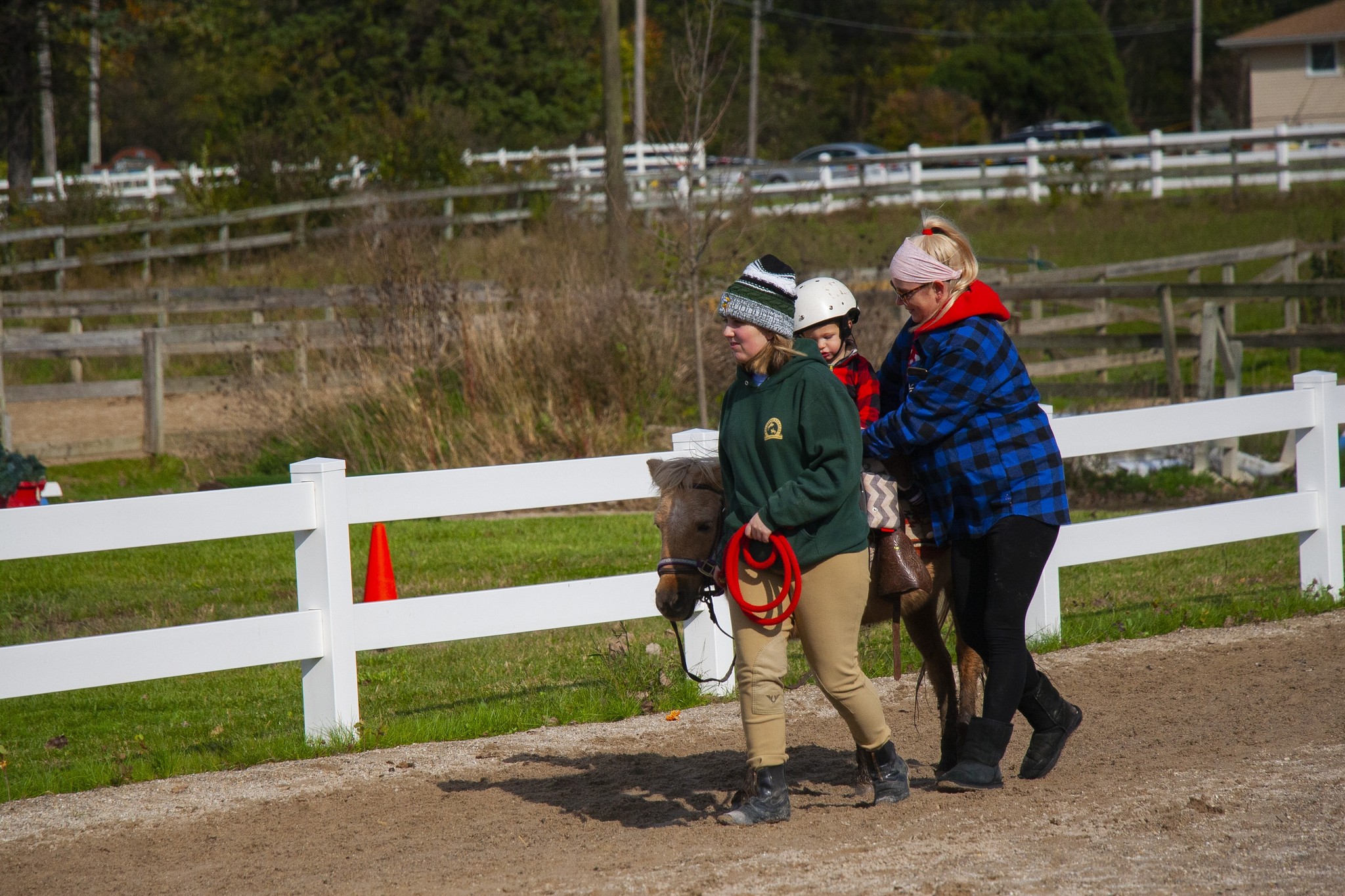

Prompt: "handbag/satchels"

[859,468,900,532]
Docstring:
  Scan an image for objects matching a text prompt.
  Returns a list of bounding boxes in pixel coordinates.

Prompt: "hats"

[717,254,797,339]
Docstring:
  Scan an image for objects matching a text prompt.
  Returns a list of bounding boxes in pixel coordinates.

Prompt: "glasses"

[890,279,934,306]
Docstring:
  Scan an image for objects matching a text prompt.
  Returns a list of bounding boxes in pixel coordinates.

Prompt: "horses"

[648,458,984,785]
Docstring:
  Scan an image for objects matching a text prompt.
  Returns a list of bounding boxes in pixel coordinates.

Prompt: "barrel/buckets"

[1,478,46,507]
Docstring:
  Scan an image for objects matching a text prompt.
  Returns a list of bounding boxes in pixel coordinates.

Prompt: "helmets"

[791,277,860,332]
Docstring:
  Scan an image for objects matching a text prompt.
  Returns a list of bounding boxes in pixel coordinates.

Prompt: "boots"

[861,739,911,806]
[716,763,790,826]
[1015,669,1083,779]
[937,716,1014,791]
[913,498,935,539]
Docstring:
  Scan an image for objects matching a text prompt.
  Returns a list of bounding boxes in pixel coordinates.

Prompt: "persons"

[716,254,910,827]
[793,277,931,597]
[863,216,1082,793]
